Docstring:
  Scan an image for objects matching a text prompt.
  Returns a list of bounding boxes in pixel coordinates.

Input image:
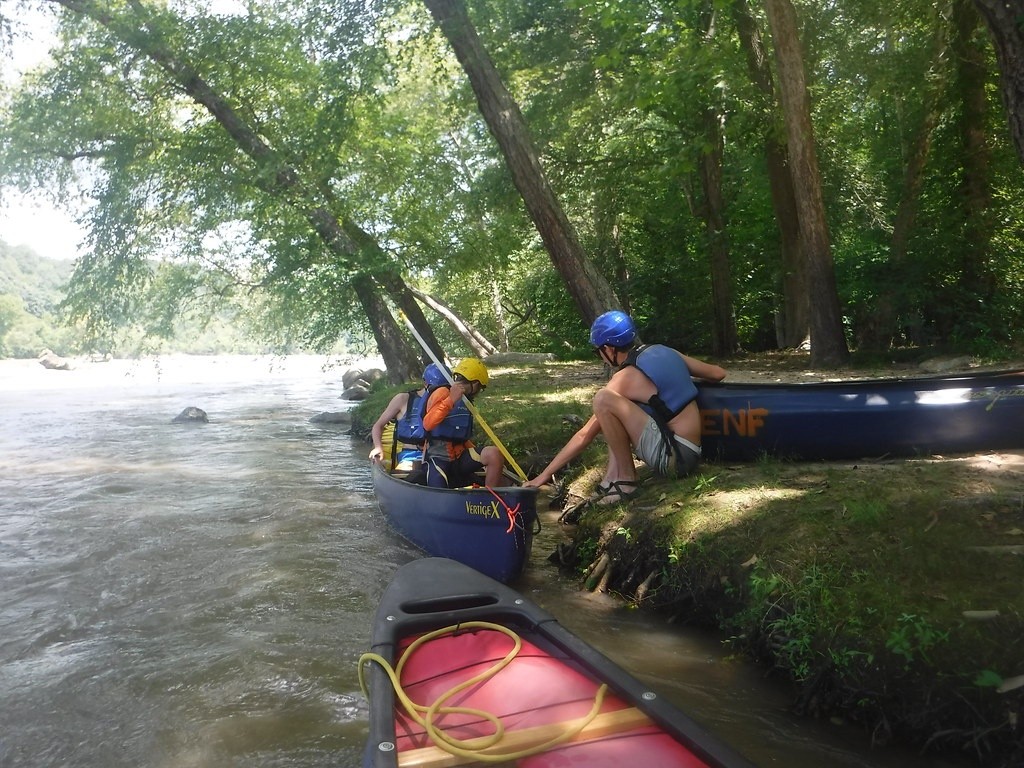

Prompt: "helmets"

[422,363,452,387]
[453,358,488,389]
[589,311,637,351]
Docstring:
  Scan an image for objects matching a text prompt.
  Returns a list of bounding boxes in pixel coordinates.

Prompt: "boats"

[368,444,540,584]
[691,370,1024,462]
[358,558,752,768]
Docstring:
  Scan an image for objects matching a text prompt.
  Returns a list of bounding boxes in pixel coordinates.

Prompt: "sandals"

[583,481,643,510]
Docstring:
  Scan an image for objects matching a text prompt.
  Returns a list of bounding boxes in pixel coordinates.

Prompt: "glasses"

[478,388,485,393]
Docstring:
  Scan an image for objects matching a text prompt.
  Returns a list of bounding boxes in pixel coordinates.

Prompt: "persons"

[522,311,725,507]
[369,363,452,478]
[410,358,505,488]
